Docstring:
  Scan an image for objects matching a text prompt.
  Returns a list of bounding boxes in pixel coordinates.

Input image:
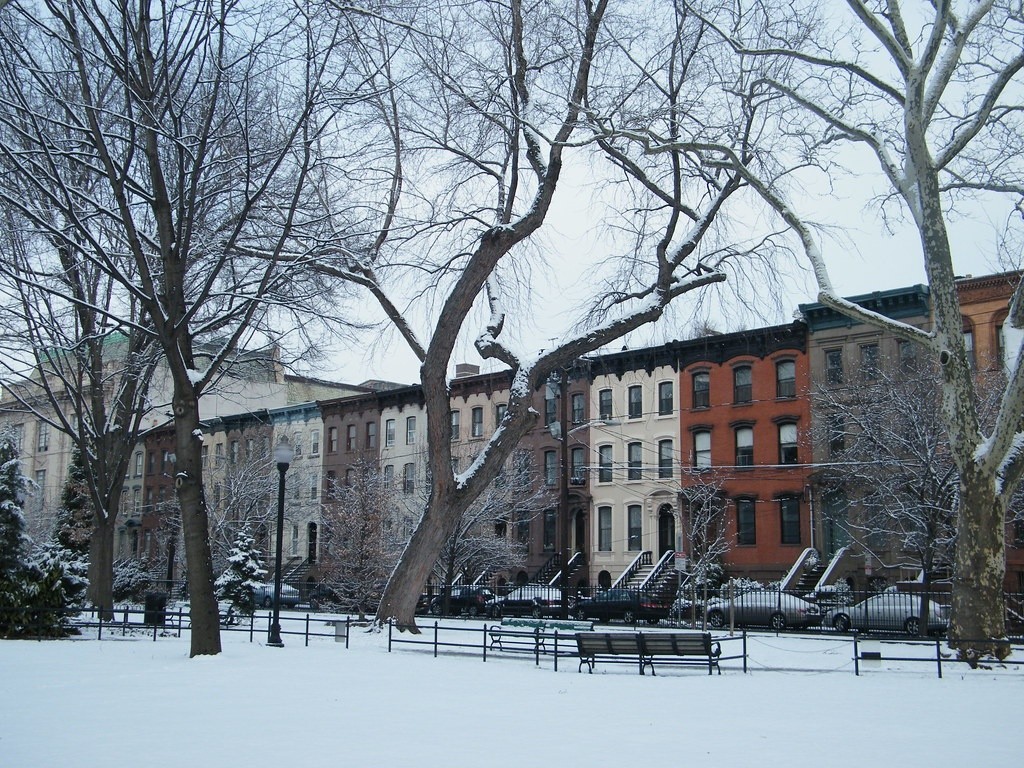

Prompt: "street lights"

[266,434,294,650]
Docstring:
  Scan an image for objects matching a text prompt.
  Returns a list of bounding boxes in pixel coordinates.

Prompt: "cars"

[483,581,567,619]
[568,587,671,626]
[247,581,300,609]
[824,593,948,635]
[429,584,496,618]
[704,588,822,632]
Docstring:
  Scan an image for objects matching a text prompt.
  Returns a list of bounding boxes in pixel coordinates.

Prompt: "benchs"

[576,631,721,676]
[489,618,595,668]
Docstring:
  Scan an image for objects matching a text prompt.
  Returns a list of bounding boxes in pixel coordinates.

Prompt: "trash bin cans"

[143,591,168,626]
